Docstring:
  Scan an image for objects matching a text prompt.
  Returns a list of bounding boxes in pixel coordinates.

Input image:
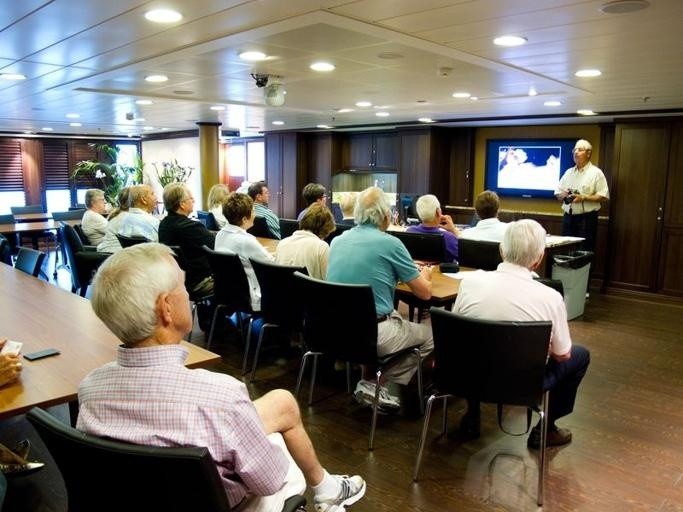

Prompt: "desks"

[0,261,221,428]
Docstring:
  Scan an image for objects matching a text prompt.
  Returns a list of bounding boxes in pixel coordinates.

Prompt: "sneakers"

[370,404,406,416]
[354,378,401,415]
[307,469,367,512]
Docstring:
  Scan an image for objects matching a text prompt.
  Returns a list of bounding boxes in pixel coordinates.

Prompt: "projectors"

[251,71,266,88]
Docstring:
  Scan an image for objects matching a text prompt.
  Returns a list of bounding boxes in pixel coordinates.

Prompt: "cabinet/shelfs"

[310,134,342,213]
[263,133,310,219]
[344,133,400,172]
[398,131,451,202]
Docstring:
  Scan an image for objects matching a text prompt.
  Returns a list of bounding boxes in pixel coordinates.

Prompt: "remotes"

[564,192,579,204]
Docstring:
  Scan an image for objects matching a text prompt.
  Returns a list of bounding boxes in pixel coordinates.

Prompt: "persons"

[81,182,590,449]
[76,243,366,512]
[1,338,24,507]
[553,138,611,299]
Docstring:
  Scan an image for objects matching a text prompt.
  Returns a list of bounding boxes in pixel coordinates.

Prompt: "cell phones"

[22,348,60,361]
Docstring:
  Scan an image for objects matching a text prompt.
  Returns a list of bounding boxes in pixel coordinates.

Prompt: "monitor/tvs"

[399,192,421,218]
[483,137,580,200]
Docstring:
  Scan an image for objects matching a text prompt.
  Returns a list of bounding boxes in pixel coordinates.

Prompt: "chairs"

[0,203,151,297]
[115,234,211,342]
[27,407,306,512]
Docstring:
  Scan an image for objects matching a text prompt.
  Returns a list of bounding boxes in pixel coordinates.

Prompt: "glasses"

[180,196,196,204]
[319,194,329,199]
[259,192,271,196]
[571,148,589,153]
[92,197,106,203]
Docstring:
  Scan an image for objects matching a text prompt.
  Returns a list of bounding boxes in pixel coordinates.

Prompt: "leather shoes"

[448,415,483,441]
[526,424,574,451]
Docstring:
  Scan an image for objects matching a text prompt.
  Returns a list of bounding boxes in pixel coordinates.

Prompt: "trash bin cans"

[551,249,594,320]
